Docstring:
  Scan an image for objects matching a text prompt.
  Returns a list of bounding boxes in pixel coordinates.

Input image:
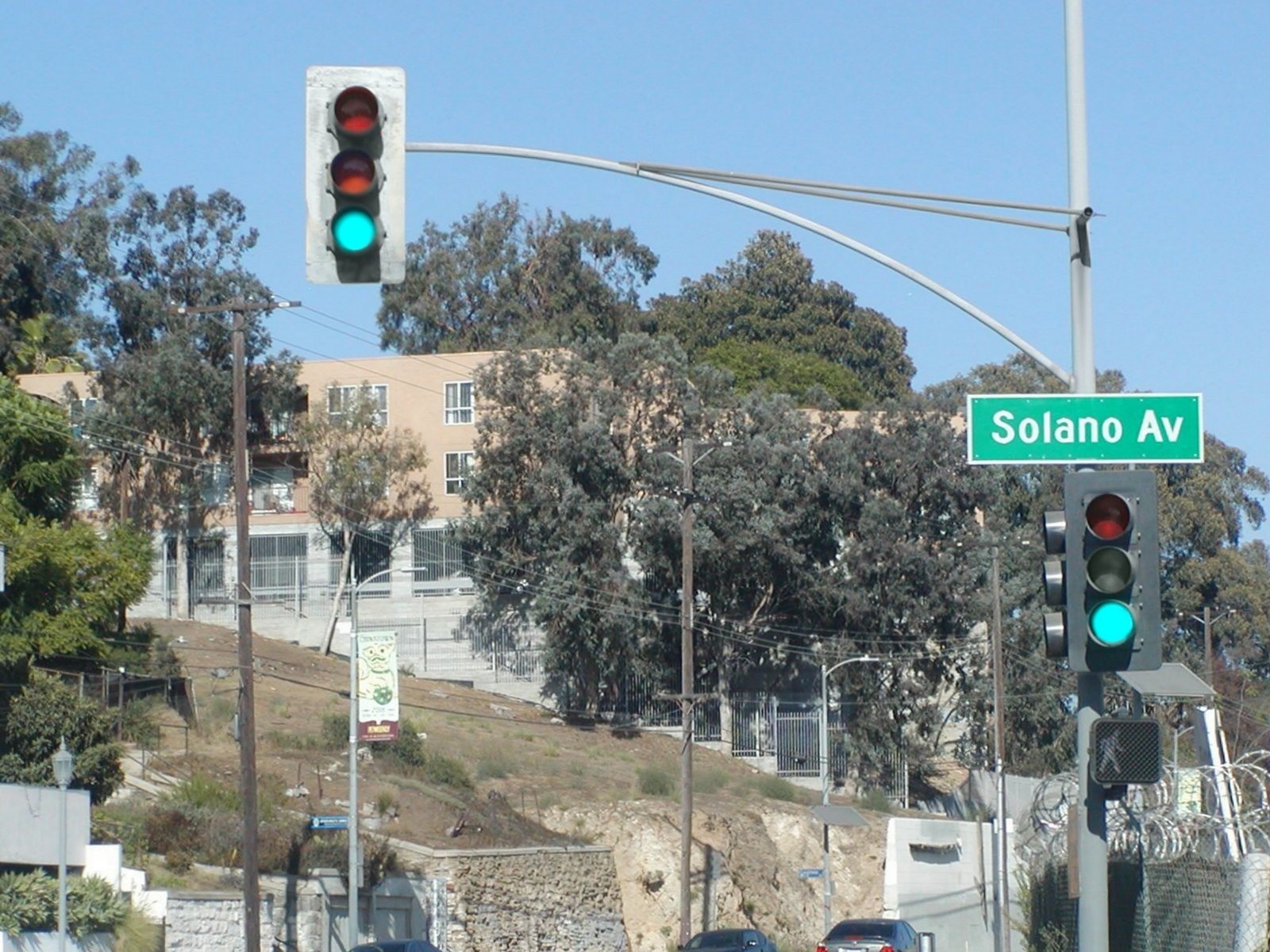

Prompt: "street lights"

[50,734,75,952]
[797,655,882,936]
[346,565,433,952]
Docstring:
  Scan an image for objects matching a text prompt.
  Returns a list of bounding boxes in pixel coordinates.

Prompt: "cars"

[814,918,935,952]
[678,928,780,952]
[347,938,442,952]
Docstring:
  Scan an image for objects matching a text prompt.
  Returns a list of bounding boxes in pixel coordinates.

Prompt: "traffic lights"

[304,66,407,287]
[1042,473,1165,672]
[1087,716,1157,786]
